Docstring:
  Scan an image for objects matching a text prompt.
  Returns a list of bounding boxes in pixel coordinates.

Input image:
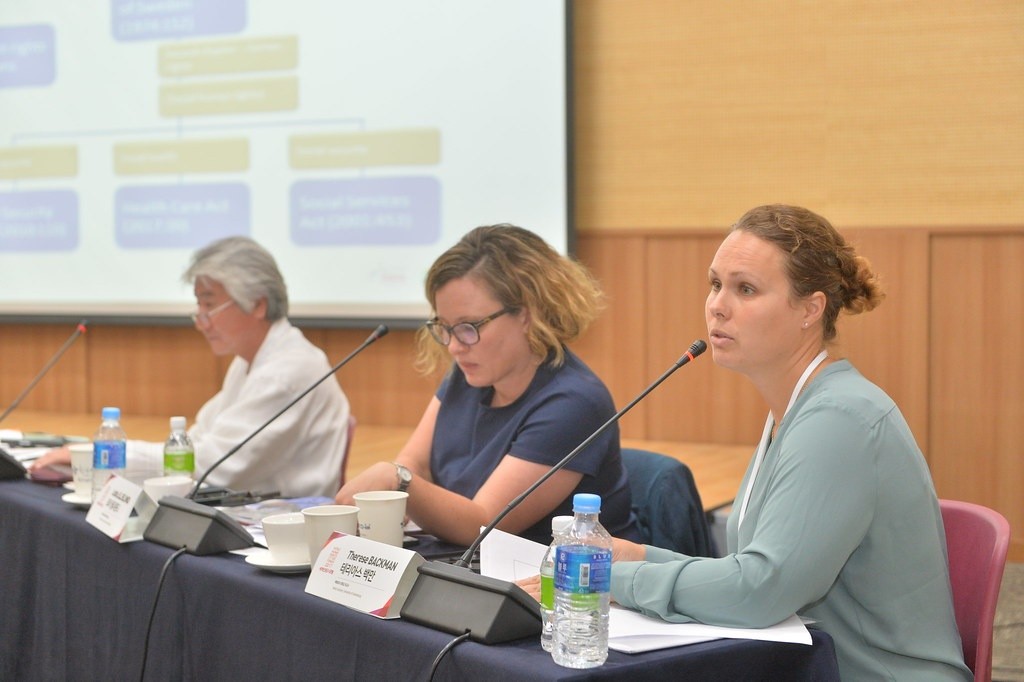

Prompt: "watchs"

[390,461,412,493]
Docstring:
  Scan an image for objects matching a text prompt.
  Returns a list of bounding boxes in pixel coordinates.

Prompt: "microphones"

[399,338,708,645]
[143,324,391,556]
[0,319,90,480]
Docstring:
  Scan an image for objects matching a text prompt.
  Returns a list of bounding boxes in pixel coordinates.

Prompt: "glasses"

[190,299,236,329]
[426,304,518,345]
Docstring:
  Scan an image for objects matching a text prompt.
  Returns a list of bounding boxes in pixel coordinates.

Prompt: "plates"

[245,553,311,574]
[61,492,96,510]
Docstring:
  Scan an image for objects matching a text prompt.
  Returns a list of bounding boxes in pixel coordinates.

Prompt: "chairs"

[936,500,1011,682]
[621,447,714,559]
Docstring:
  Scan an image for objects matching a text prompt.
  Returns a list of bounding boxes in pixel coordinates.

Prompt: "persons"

[510,206,975,682]
[31,236,349,500]
[332,222,644,549]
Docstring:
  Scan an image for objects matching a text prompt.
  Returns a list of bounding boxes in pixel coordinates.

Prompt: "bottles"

[93,406,127,503]
[540,516,574,653]
[164,416,196,474]
[551,494,612,669]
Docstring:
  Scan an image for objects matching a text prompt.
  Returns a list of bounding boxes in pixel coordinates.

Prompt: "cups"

[70,444,94,500]
[352,490,410,548]
[261,514,313,563]
[302,505,360,573]
[144,476,193,507]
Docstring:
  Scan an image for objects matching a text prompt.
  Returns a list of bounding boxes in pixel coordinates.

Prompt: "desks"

[0,479,842,682]
